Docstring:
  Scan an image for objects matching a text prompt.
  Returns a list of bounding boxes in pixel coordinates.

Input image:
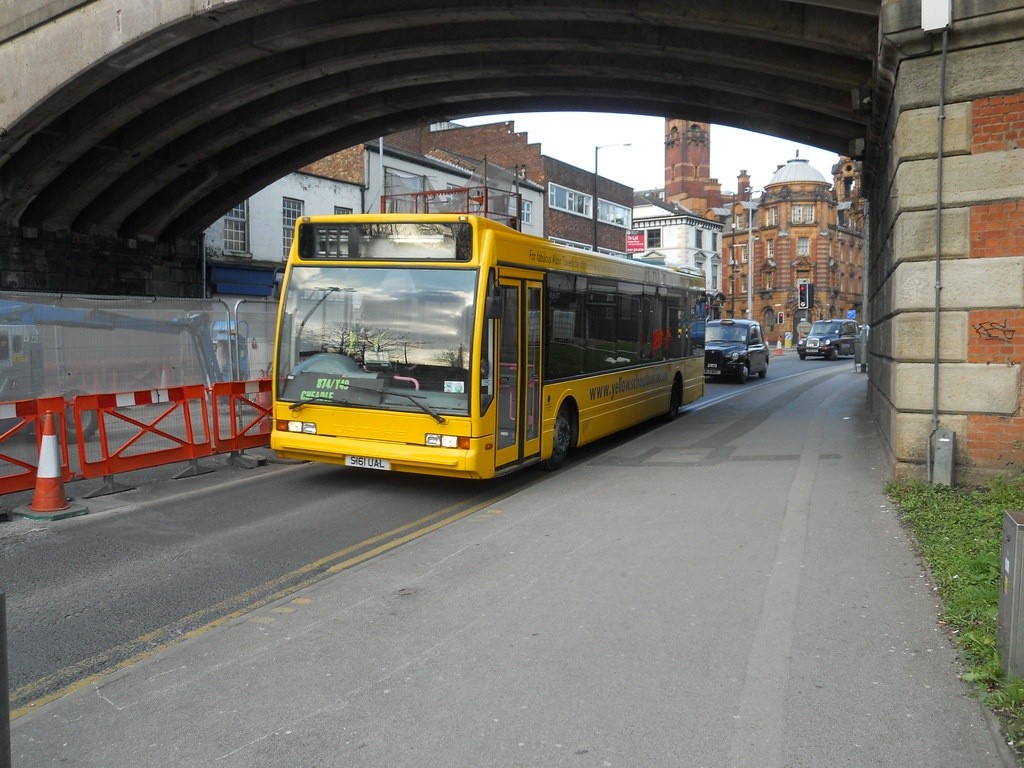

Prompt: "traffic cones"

[7,410,91,522]
[773,335,786,357]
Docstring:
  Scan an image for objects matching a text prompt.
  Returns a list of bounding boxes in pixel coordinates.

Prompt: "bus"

[265,210,710,478]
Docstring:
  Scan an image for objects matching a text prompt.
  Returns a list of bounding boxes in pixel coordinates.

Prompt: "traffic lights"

[797,278,811,310]
[778,312,784,325]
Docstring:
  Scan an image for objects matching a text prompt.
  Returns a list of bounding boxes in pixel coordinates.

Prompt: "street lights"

[743,186,763,320]
[595,142,632,252]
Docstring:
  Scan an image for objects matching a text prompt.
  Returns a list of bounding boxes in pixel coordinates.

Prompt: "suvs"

[797,319,860,360]
[704,319,770,385]
